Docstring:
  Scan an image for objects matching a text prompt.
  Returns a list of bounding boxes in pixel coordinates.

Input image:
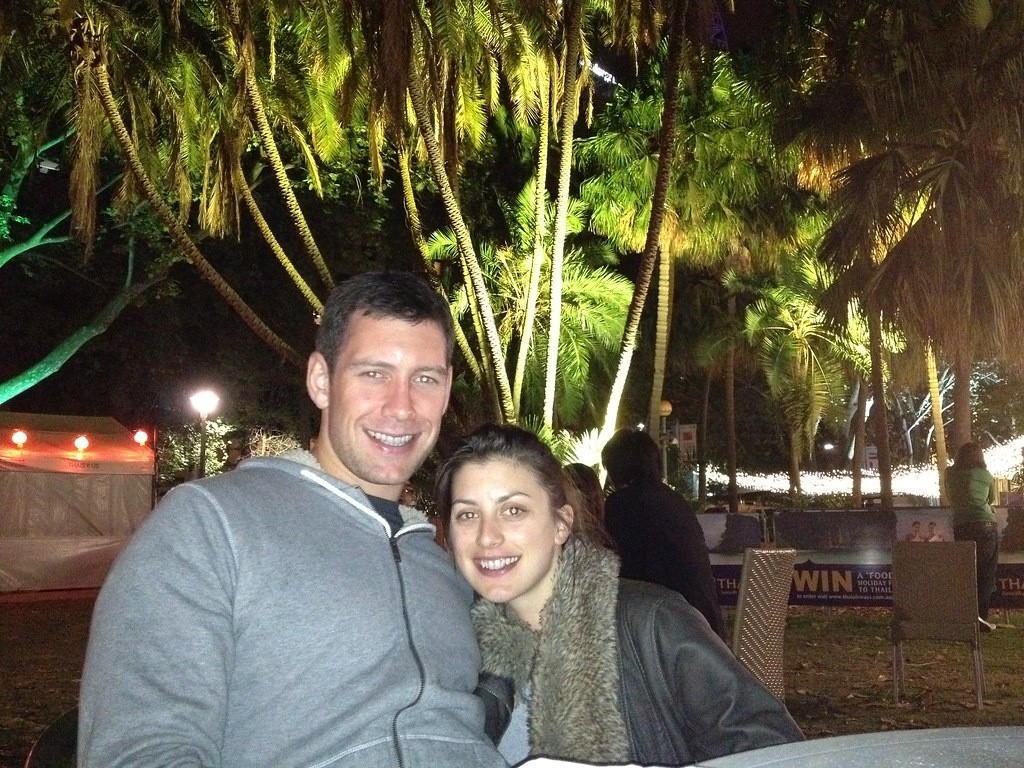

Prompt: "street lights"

[191,389,220,478]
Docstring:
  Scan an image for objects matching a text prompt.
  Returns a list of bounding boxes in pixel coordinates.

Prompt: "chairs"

[889,540,998,710]
[732,547,796,704]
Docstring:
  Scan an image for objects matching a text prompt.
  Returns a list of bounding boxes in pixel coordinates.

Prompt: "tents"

[0,412,155,601]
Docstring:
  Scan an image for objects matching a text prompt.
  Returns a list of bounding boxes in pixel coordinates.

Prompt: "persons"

[600,427,727,642]
[431,424,808,768]
[905,521,944,542]
[78,275,510,767]
[943,442,1000,631]
[563,462,615,550]
